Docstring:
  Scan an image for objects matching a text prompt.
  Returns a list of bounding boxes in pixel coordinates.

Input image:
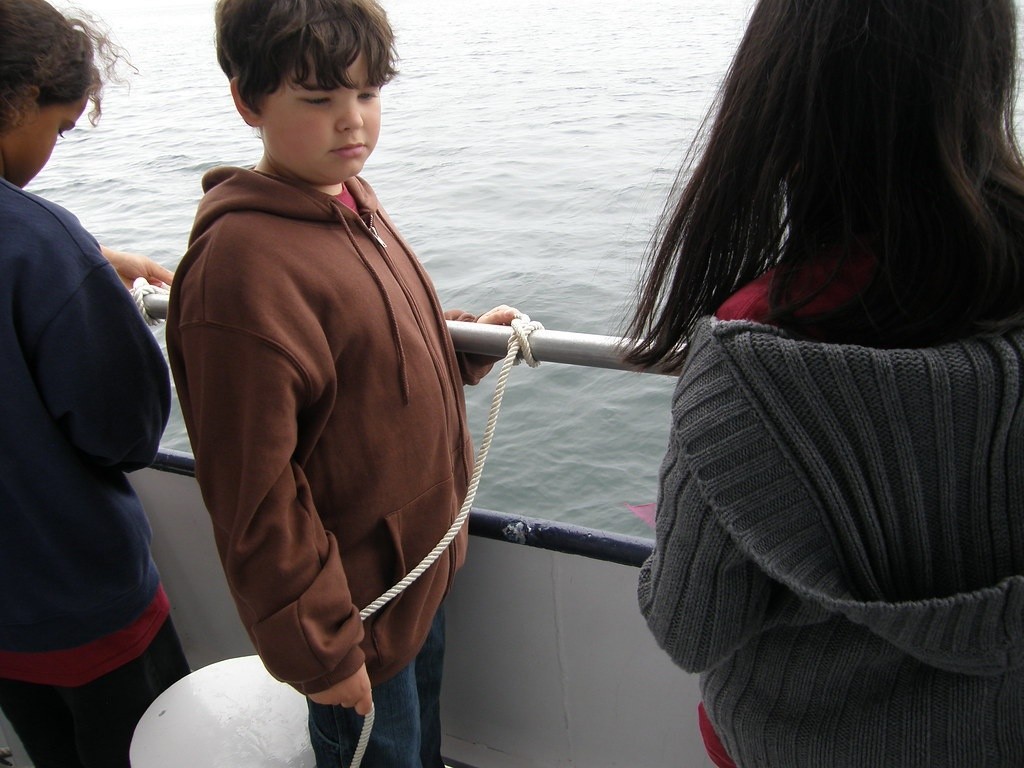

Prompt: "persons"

[162,0,533,768]
[0,0,200,768]
[617,0,1024,768]
